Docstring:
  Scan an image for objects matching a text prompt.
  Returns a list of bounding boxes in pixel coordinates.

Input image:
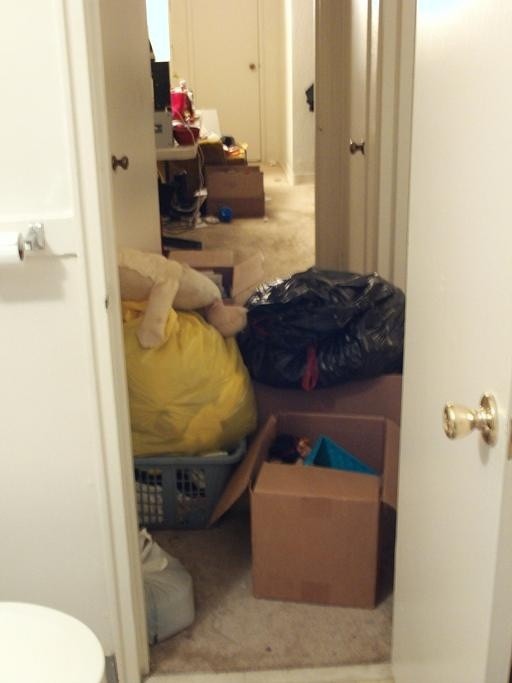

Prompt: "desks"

[155,145,200,159]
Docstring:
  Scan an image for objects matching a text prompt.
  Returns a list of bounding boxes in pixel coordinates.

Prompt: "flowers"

[176,111,200,129]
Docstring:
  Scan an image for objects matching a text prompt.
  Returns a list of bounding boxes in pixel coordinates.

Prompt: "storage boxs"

[206,412,400,608]
[203,167,265,218]
[256,374,399,419]
